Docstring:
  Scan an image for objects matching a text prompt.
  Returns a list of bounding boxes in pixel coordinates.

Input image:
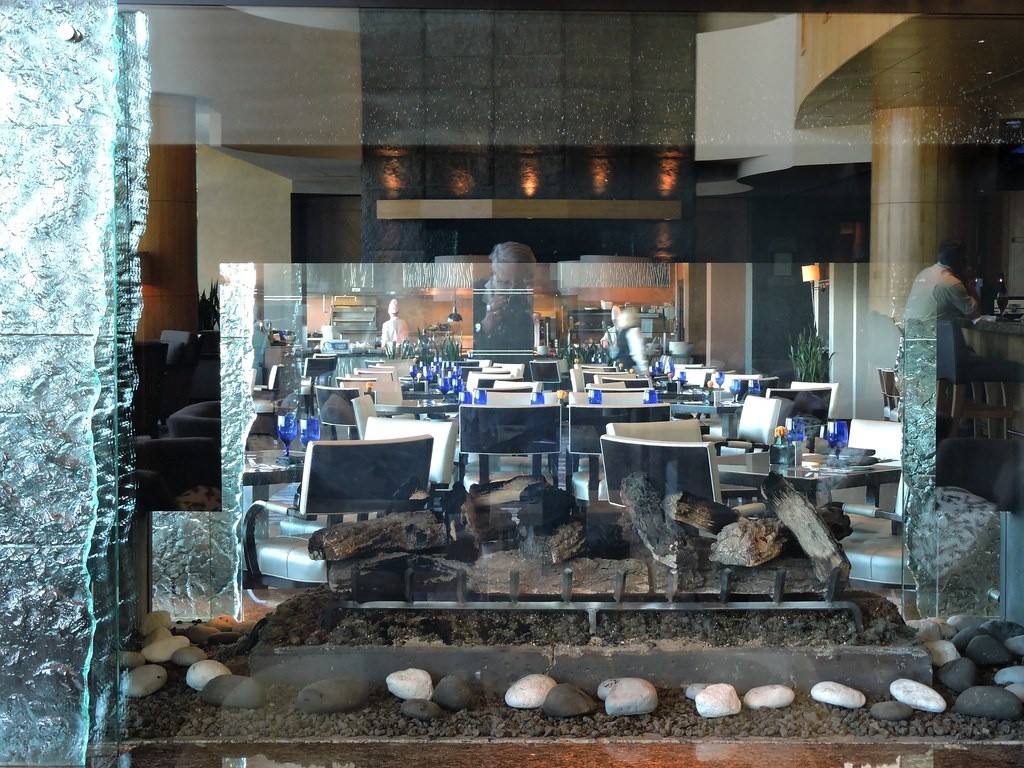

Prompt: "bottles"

[364,382,375,403]
[649,358,663,375]
[678,367,687,395]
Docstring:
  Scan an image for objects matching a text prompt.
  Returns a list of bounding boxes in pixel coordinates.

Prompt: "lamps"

[801,265,830,293]
[446,288,462,321]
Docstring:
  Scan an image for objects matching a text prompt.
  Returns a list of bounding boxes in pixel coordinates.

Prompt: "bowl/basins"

[536,345,549,354]
[600,299,640,312]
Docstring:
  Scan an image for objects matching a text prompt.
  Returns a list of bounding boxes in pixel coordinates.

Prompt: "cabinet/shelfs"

[568,309,666,355]
[330,296,379,335]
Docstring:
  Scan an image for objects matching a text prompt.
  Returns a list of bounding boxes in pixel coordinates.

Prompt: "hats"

[611,306,620,320]
[388,299,399,313]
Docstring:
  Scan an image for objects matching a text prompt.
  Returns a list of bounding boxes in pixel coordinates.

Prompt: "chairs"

[134,328,222,512]
[238,352,916,592]
[936,320,1024,440]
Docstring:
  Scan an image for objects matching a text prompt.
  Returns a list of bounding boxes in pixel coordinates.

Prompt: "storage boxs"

[769,444,795,466]
[367,348,383,354]
[656,309,663,313]
[650,305,657,309]
[976,272,996,316]
[574,324,586,329]
[323,339,351,355]
[648,308,656,314]
[668,383,677,392]
[584,337,594,344]
[659,305,664,309]
[601,300,613,311]
[413,382,425,391]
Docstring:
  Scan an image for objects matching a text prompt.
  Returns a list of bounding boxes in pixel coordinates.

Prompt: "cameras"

[500,289,528,313]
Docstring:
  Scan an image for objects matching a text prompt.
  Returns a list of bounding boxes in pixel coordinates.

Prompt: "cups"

[458,392,472,406]
[643,390,657,403]
[530,392,544,404]
[588,390,602,404]
[297,419,320,447]
[669,341,688,354]
[748,380,760,391]
[474,389,487,405]
[285,330,295,335]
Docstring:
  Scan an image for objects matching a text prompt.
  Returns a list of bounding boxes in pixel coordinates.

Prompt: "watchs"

[474,322,488,337]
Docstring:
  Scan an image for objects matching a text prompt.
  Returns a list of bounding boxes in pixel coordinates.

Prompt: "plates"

[584,307,598,310]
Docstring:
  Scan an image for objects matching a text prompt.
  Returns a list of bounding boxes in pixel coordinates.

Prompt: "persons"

[473,241,538,364]
[381,299,409,347]
[319,390,356,425]
[600,306,650,376]
[896,239,983,523]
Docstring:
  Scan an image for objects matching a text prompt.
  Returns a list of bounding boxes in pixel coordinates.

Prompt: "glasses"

[494,272,537,290]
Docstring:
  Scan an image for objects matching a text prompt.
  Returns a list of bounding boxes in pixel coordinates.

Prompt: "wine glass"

[784,417,803,445]
[278,415,297,456]
[730,379,740,402]
[716,372,725,388]
[409,357,464,402]
[590,353,607,364]
[827,422,848,470]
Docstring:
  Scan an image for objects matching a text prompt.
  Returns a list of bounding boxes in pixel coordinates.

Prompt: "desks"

[671,402,744,436]
[375,398,461,420]
[403,390,458,399]
[401,383,438,388]
[718,459,902,505]
[245,449,306,542]
[659,393,706,401]
[654,375,668,382]
[458,361,479,367]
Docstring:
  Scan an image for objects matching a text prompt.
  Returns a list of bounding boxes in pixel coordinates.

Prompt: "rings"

[494,310,497,316]
